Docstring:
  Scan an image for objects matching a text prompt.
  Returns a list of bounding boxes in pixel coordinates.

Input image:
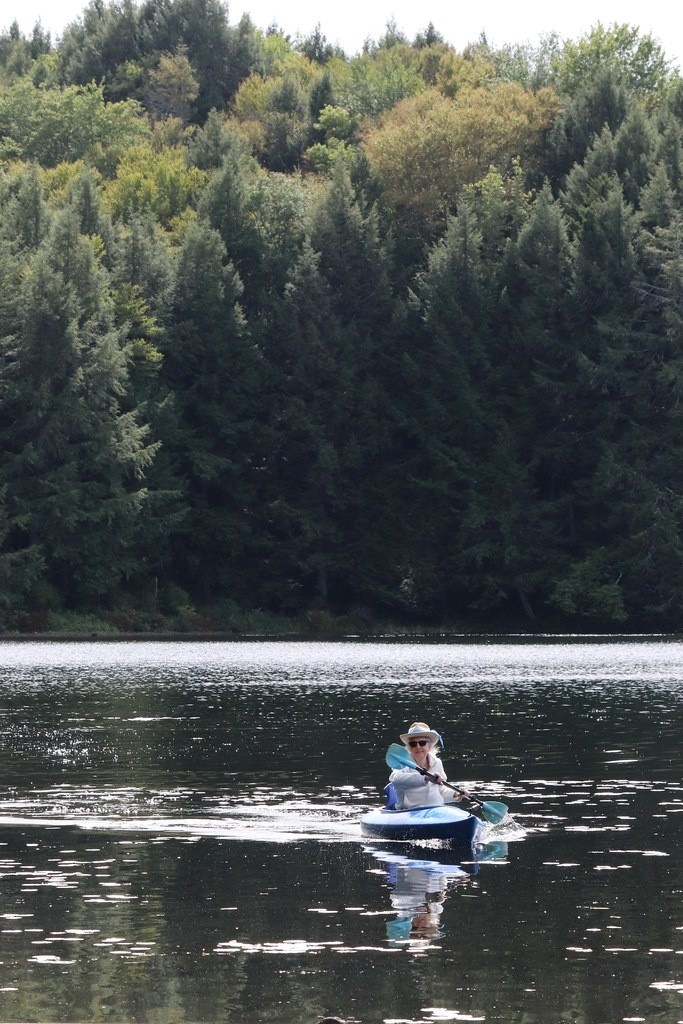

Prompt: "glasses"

[409,741,426,747]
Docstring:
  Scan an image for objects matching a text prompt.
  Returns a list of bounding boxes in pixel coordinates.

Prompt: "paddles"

[385,742,509,824]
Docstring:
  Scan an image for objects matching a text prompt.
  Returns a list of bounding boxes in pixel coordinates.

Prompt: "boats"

[361,838,482,875]
[359,806,483,852]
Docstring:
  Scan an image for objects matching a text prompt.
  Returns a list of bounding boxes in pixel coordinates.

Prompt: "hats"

[400,723,439,745]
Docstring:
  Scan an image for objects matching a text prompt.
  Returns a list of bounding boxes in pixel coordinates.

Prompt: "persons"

[389,722,469,808]
